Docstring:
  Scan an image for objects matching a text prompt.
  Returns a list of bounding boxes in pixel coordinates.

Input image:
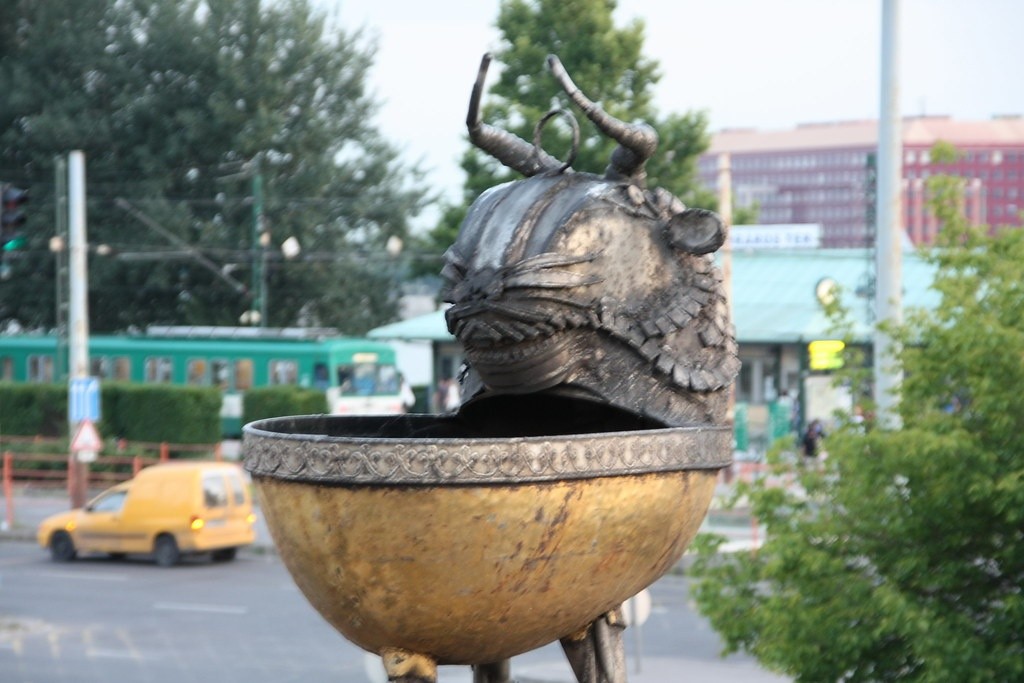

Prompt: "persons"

[846,405,865,435]
[396,373,417,417]
[434,376,463,412]
[777,391,792,406]
[803,420,826,456]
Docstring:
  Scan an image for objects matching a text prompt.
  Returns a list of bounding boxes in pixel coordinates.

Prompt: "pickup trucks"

[36,459,255,567]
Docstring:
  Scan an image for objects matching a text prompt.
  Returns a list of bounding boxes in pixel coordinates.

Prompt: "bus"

[0,325,410,439]
